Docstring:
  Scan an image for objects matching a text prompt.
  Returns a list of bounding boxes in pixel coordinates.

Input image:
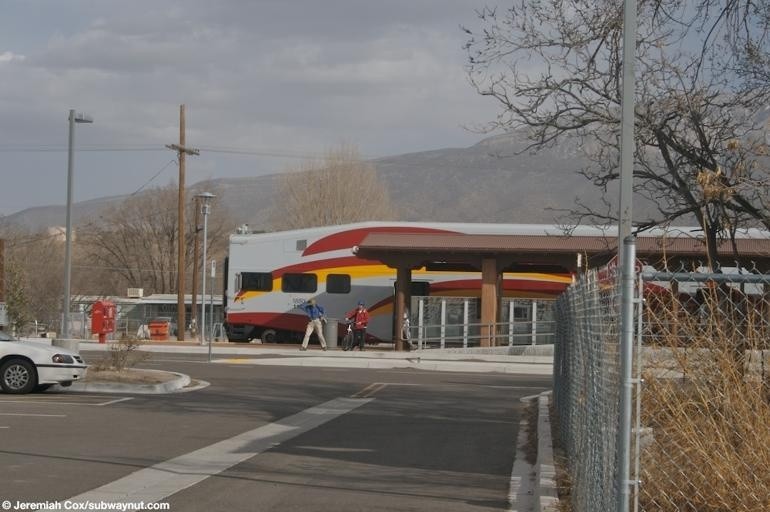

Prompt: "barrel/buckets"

[322,318,339,350]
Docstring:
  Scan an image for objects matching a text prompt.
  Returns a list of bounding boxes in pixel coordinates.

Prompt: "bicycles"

[341,319,355,350]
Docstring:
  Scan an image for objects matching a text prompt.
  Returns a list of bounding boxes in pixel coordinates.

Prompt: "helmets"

[357,300,365,305]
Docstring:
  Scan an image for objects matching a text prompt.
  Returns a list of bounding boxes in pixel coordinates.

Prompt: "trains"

[222,220,769,351]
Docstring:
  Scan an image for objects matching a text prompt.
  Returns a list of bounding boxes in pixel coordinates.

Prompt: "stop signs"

[608,249,642,283]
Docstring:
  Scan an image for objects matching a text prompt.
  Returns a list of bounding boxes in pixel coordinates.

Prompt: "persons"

[345,299,369,351]
[298,299,328,351]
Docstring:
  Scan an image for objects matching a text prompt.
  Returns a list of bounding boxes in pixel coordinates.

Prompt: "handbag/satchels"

[321,316,329,327]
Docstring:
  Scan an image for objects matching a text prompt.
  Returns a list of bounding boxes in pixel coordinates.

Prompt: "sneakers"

[298,347,328,351]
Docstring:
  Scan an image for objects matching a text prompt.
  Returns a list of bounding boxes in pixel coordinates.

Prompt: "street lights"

[194,192,216,344]
[62,108,93,335]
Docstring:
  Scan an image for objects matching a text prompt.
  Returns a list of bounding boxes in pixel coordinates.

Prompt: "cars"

[0,329,86,394]
